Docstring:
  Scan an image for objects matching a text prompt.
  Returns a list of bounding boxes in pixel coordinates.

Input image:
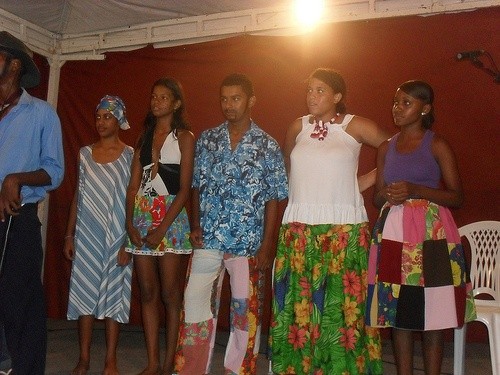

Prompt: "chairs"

[454,220,500,375]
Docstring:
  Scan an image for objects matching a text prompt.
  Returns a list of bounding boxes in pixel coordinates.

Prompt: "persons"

[170,72,289,375]
[269,67,391,375]
[63,94,136,375]
[373,80,478,375]
[0,30,65,375]
[125,77,198,375]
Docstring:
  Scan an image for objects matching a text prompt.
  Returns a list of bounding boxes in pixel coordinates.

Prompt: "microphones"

[456,50,484,62]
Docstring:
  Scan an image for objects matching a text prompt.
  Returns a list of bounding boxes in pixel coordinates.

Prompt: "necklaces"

[310,112,340,141]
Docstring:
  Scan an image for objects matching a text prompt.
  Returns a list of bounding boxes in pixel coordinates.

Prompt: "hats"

[0,30,40,88]
[96,95,130,131]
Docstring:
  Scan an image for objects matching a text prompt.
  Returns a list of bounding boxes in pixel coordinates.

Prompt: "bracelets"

[64,236,75,239]
